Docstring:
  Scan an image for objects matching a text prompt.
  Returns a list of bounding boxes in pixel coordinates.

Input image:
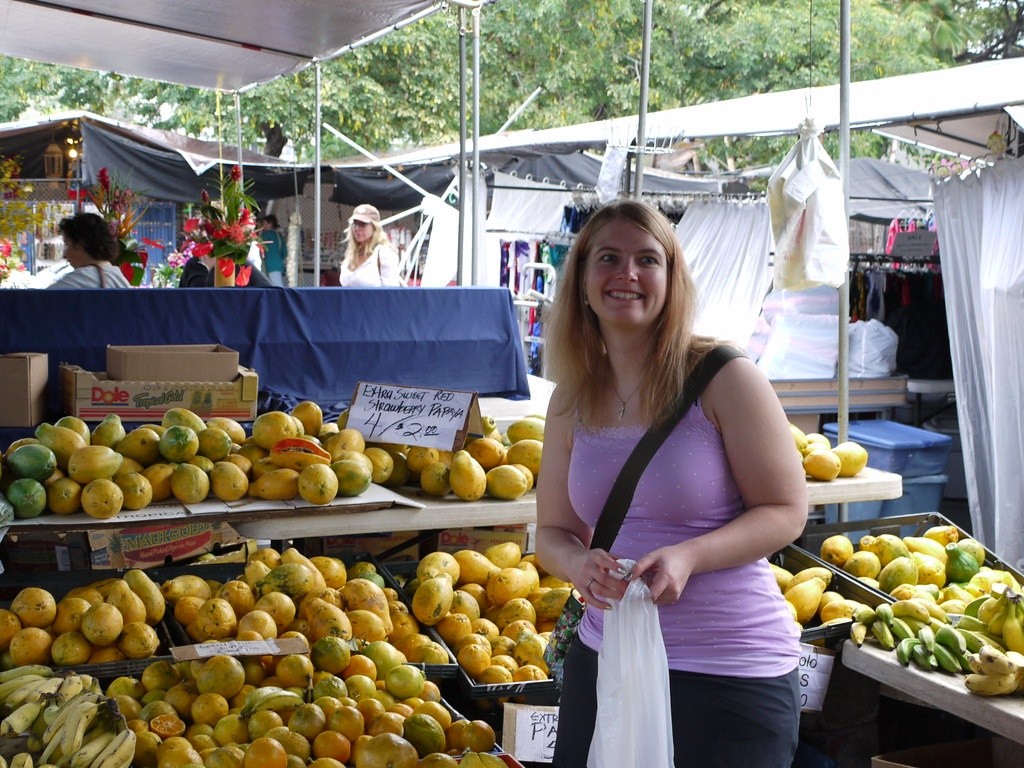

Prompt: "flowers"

[79,164,165,281]
[180,166,274,286]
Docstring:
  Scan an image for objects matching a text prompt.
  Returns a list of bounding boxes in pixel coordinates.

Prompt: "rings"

[586,577,594,590]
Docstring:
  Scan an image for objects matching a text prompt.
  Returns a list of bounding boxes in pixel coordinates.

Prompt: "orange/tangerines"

[99,631,511,768]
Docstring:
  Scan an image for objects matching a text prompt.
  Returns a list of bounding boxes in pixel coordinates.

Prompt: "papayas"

[402,540,575,685]
[787,420,868,482]
[161,547,450,663]
[0,399,541,519]
[765,524,1023,623]
[0,565,167,670]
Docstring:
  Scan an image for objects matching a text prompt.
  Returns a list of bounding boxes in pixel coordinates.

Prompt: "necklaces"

[605,348,653,421]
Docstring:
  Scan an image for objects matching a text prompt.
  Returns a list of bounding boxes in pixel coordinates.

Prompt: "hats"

[347,203,381,223]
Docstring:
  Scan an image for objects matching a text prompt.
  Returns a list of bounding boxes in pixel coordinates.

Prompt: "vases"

[214,258,235,287]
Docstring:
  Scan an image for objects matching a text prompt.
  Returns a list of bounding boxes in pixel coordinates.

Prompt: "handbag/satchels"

[848,318,899,378]
[541,586,586,693]
[377,247,408,288]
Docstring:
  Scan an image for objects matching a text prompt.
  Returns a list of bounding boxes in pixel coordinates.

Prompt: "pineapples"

[109,530,126,568]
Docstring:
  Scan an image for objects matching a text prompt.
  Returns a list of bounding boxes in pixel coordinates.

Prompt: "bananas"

[0,663,137,768]
[851,584,1024,695]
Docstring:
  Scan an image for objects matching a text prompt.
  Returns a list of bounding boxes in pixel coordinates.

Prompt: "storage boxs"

[0,344,1024,768]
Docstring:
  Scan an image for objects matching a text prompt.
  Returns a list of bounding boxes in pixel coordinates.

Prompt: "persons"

[178,241,268,288]
[533,200,809,768]
[45,214,132,288]
[258,215,287,288]
[340,204,400,289]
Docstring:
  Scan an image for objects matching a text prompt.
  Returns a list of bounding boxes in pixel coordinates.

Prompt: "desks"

[842,638,1024,746]
[906,379,954,427]
[228,466,902,561]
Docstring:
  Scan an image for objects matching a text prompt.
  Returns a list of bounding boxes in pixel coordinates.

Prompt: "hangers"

[564,188,769,211]
[859,256,932,277]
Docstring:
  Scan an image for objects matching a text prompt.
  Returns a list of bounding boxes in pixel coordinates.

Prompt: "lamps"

[43,143,63,189]
